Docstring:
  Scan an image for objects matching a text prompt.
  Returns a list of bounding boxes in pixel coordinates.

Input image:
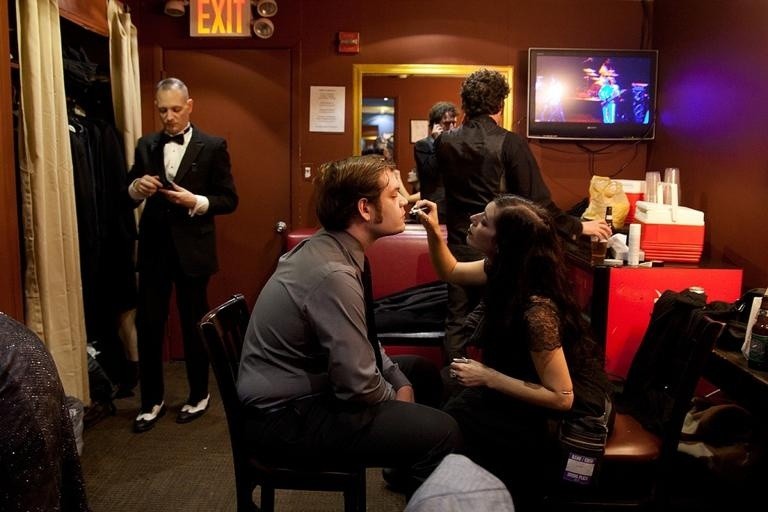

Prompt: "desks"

[702,345,767,420]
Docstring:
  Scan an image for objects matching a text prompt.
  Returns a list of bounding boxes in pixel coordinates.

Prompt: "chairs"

[199,295,366,511]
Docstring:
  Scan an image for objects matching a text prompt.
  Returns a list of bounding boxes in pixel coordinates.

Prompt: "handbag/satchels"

[581,175,630,229]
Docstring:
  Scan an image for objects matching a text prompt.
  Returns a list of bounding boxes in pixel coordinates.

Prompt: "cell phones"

[431,120,443,135]
[156,176,173,191]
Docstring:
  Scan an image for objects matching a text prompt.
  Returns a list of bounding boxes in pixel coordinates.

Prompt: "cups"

[644,167,682,204]
[589,234,607,260]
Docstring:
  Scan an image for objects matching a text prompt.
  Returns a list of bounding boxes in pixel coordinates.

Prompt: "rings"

[449,369,456,379]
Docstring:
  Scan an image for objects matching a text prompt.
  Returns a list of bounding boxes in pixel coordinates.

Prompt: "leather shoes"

[176,391,210,423]
[133,401,165,431]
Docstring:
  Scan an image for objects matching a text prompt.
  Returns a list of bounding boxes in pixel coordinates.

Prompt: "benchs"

[284,227,484,379]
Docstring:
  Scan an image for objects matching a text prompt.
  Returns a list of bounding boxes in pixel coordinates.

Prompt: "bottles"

[605,206,615,234]
[628,224,641,267]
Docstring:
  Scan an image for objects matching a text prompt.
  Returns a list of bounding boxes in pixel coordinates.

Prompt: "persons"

[361,136,394,155]
[66,75,239,434]
[596,67,623,125]
[235,155,462,502]
[0,307,87,512]
[415,102,465,218]
[399,188,617,511]
[435,67,616,263]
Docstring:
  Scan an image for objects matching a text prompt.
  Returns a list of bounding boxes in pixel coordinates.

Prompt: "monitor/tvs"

[525,45,660,143]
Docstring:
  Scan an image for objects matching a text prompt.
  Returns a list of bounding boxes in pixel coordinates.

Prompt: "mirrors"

[352,61,515,226]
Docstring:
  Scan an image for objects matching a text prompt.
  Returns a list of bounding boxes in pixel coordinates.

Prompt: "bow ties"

[161,133,184,145]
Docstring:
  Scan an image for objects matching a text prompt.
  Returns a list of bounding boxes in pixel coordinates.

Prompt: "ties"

[362,255,383,372]
